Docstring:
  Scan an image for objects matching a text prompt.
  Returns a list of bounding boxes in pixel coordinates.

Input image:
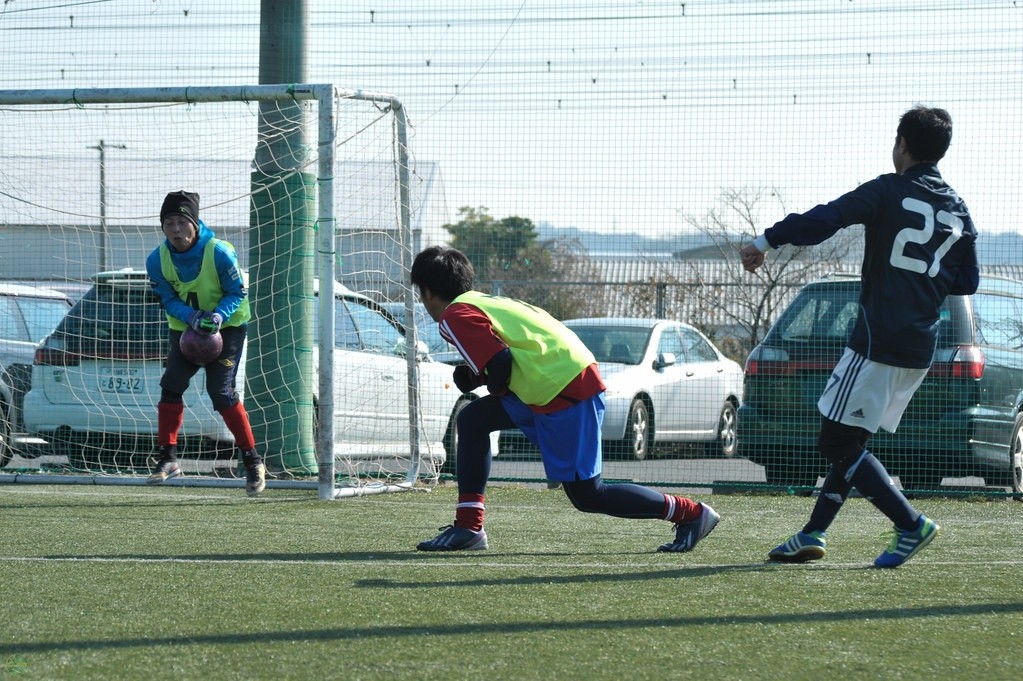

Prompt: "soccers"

[180,326,224,366]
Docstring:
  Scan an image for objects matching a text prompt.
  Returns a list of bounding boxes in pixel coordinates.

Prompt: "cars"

[0,283,76,466]
[38,285,91,302]
[561,317,744,459]
[378,301,466,365]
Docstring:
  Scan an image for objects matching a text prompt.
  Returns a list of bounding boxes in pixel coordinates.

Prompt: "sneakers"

[145,461,181,486]
[768,529,828,562]
[873,511,941,569]
[243,462,266,496]
[416,519,490,550]
[656,501,721,553]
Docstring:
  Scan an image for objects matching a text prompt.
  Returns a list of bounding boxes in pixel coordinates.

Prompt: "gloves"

[452,364,482,394]
[487,386,511,397]
[193,310,224,336]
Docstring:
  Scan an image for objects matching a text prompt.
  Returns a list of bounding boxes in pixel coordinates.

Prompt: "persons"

[410,244,721,553]
[145,190,266,497]
[743,107,979,568]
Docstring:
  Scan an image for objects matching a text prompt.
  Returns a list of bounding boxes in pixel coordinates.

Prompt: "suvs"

[24,275,501,476]
[736,269,1023,501]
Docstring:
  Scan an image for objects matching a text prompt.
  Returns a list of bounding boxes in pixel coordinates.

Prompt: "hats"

[159,190,200,231]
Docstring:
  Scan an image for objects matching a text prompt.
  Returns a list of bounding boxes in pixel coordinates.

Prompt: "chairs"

[610,343,635,363]
